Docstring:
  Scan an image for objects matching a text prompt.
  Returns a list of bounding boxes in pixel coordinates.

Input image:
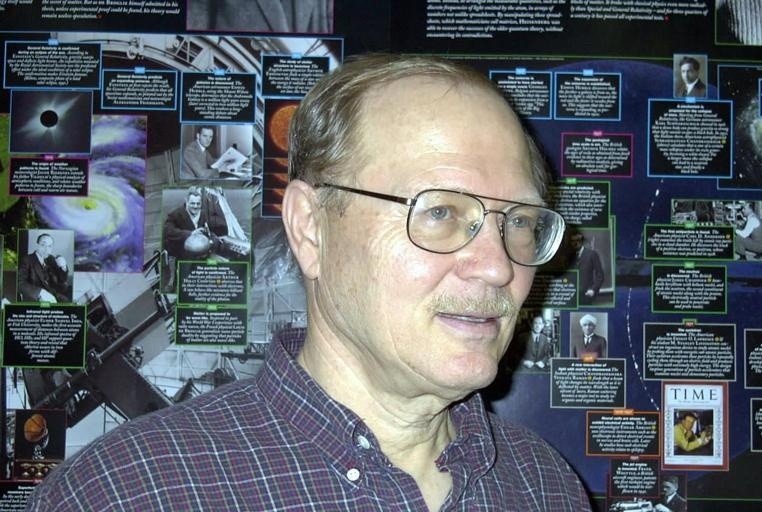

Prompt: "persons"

[184,123,227,179]
[17,234,69,305]
[734,198,761,259]
[572,226,605,308]
[516,314,551,369]
[570,315,607,359]
[676,56,705,98]
[23,49,596,512]
[162,186,222,262]
[676,409,712,456]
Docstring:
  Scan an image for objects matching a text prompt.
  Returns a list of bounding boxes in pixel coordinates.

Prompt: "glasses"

[313,181,566,266]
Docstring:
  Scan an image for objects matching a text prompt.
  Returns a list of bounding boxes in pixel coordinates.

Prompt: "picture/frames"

[660,376,729,473]
